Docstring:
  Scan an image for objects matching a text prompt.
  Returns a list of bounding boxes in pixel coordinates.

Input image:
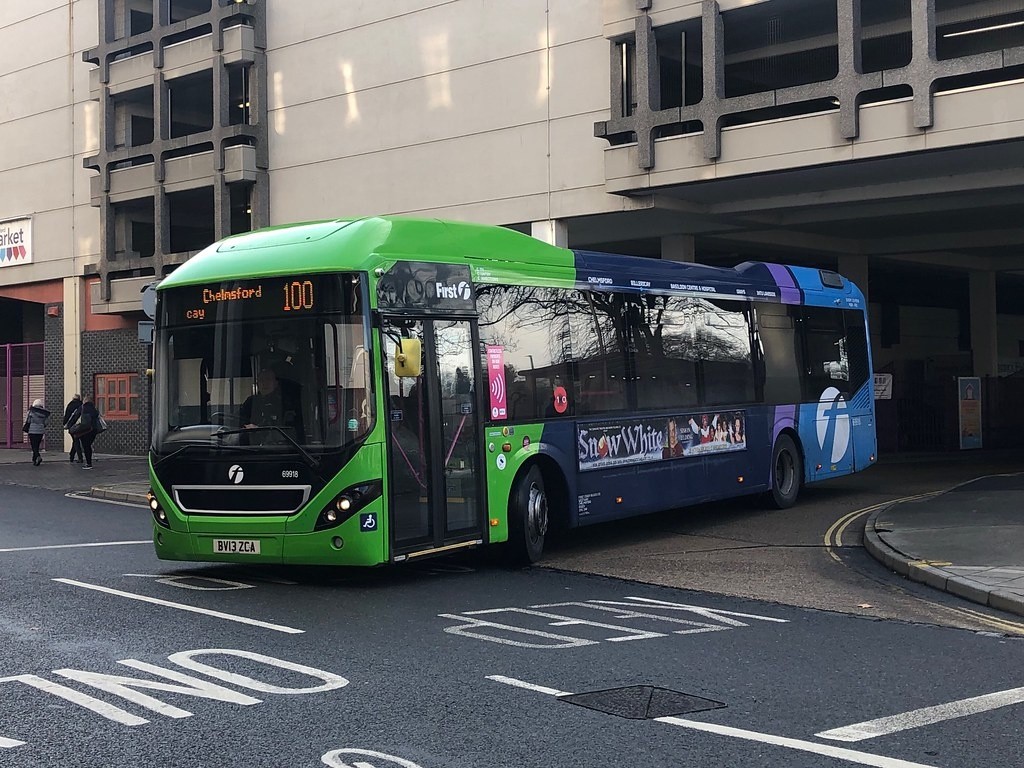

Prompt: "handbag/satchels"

[97,416,108,433]
[64,423,68,429]
[22,417,31,433]
[69,413,93,438]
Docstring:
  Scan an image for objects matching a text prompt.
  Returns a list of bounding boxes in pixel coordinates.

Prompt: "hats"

[32,399,41,407]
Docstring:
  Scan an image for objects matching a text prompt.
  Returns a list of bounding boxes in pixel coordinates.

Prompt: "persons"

[238,368,296,444]
[25,398,50,466]
[71,396,99,470]
[63,393,84,463]
[660,411,745,459]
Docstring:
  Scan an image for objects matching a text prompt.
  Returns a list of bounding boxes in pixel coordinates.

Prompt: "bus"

[143,212,882,571]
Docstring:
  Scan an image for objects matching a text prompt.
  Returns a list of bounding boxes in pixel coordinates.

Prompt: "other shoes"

[82,464,93,470]
[70,459,74,462]
[36,458,42,466]
[76,459,83,463]
